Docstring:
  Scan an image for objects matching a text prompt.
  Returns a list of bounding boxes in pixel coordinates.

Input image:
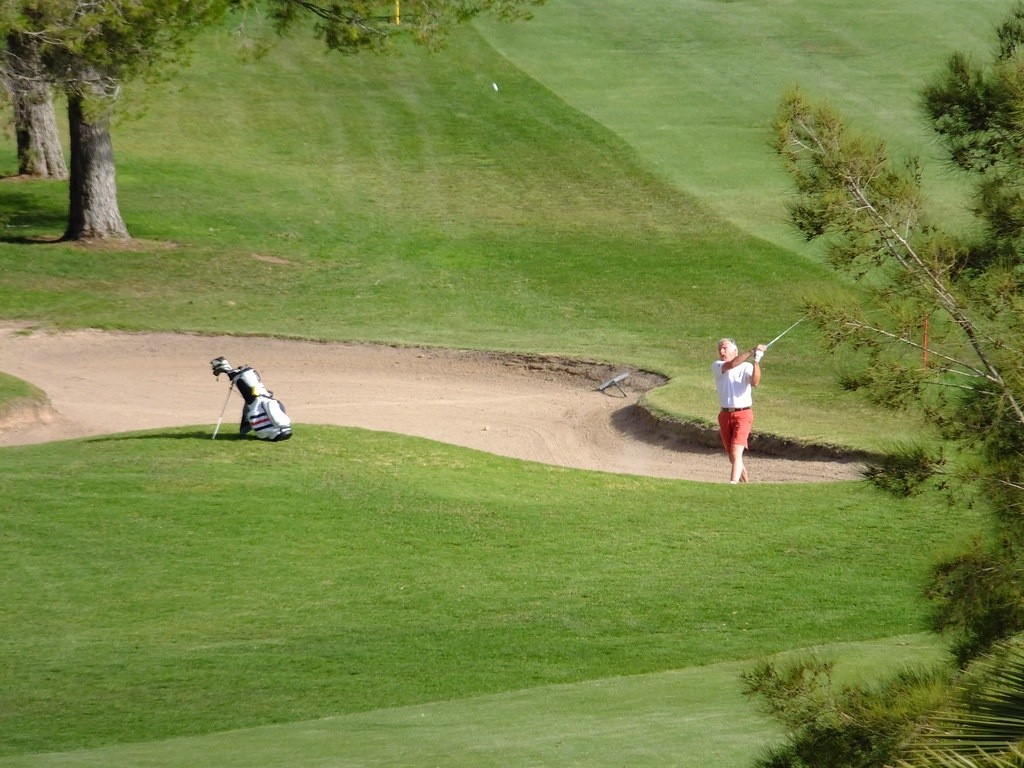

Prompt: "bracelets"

[749,350,754,354]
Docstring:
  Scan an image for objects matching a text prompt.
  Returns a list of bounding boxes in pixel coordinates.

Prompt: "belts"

[722,407,751,411]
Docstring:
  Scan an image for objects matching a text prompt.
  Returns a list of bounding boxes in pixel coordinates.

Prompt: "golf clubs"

[766,314,806,348]
[210,356,233,377]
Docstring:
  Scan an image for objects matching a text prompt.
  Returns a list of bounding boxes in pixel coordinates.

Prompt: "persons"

[711,339,767,485]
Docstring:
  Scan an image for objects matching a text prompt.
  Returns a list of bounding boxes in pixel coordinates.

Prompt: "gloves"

[754,350,764,362]
